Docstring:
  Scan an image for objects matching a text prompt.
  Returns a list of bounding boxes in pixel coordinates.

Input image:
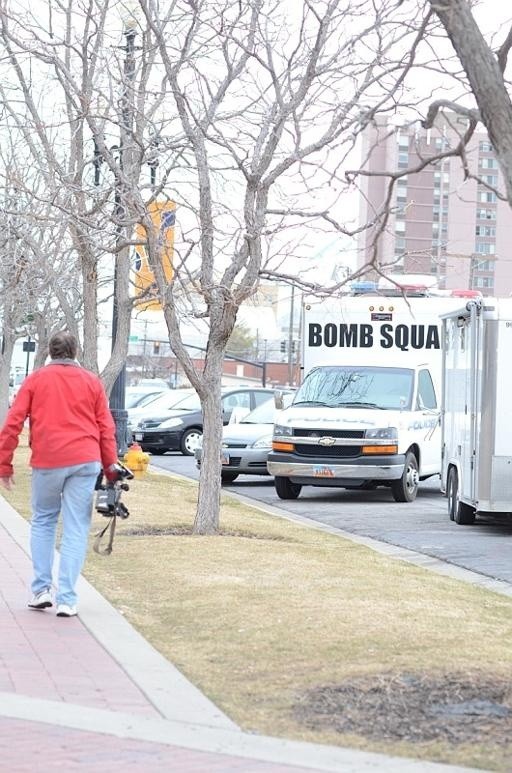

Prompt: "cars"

[125,387,295,482]
[8,371,32,407]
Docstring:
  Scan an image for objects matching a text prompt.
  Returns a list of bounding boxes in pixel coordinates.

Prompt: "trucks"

[267,275,482,502]
[439,294,512,525]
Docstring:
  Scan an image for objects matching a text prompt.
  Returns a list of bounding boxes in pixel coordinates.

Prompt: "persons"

[0,330,126,618]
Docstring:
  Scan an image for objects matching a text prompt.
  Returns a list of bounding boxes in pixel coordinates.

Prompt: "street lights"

[92,0,157,457]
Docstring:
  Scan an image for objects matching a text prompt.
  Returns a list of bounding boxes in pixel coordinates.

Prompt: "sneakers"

[27,590,78,616]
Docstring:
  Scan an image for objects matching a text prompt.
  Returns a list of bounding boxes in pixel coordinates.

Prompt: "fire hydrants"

[123,441,150,471]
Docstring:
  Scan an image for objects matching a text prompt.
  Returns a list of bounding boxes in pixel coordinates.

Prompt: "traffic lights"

[280,340,296,354]
[154,341,160,354]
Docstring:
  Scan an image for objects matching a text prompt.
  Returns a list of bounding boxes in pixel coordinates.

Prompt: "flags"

[132,201,181,310]
